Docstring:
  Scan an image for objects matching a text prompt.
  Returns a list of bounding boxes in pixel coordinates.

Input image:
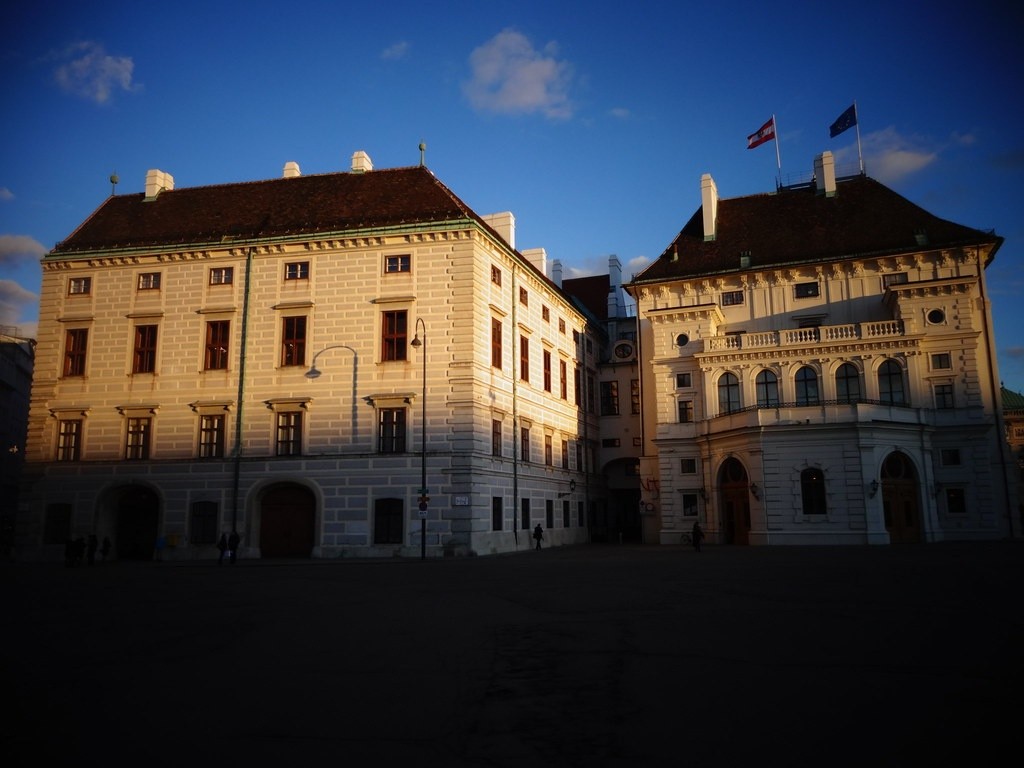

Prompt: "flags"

[829,104,857,138]
[747,116,775,149]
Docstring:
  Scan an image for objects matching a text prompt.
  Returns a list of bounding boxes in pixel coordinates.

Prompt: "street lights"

[409,316,428,560]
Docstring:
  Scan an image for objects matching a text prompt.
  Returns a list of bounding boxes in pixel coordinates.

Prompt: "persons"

[535,524,544,553]
[217,529,240,564]
[692,522,705,552]
[615,526,626,545]
[0,524,111,569]
